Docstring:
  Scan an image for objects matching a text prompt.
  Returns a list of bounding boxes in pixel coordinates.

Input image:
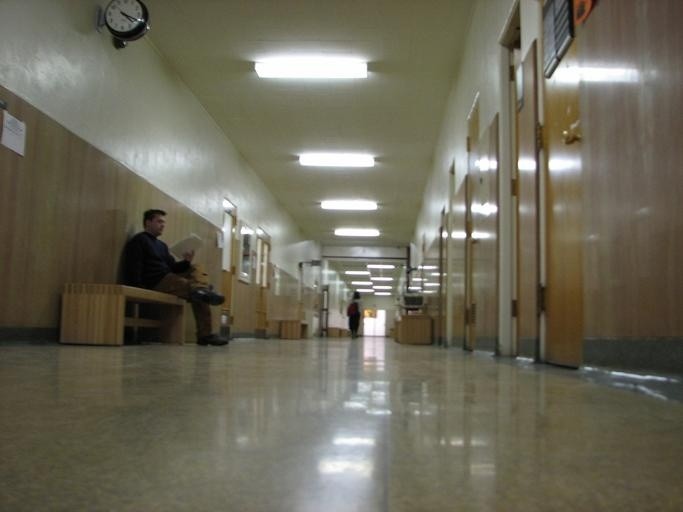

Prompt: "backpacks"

[347,303,358,315]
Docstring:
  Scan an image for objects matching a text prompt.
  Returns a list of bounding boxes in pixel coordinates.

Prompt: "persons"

[346,291,362,338]
[123,209,229,346]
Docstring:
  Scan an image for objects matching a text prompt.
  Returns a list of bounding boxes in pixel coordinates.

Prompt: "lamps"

[251,56,382,238]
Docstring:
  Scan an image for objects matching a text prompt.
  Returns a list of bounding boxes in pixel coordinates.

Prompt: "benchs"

[59,281,187,346]
[280,320,308,339]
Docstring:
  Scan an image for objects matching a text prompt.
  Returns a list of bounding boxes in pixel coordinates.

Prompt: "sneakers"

[195,288,225,305]
[197,335,228,345]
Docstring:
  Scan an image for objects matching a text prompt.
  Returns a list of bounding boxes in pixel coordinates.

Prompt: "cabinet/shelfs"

[328,327,348,336]
[395,314,433,345]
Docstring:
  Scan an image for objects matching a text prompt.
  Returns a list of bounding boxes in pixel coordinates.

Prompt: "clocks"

[95,0,151,49]
